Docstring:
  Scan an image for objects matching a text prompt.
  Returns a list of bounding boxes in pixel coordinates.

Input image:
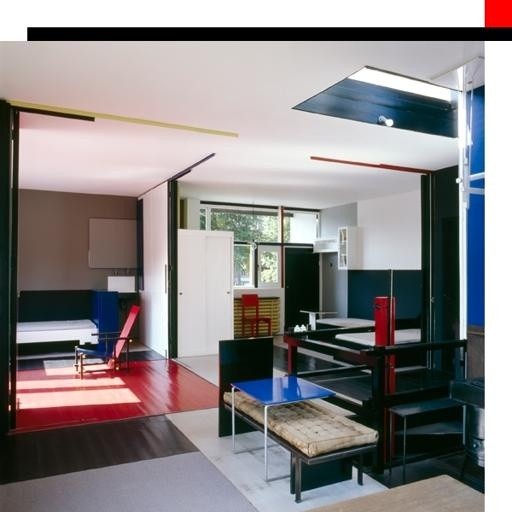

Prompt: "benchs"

[225,391,379,505]
[390,398,467,466]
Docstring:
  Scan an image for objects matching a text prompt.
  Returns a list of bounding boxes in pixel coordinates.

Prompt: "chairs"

[74,302,142,379]
[241,293,271,336]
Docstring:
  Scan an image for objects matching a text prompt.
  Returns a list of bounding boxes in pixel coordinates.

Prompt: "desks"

[230,376,336,482]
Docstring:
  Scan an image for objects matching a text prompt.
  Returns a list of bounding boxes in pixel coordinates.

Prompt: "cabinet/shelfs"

[178,228,234,357]
[337,226,361,271]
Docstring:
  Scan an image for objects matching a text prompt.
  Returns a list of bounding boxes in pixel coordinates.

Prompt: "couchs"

[15,290,97,361]
